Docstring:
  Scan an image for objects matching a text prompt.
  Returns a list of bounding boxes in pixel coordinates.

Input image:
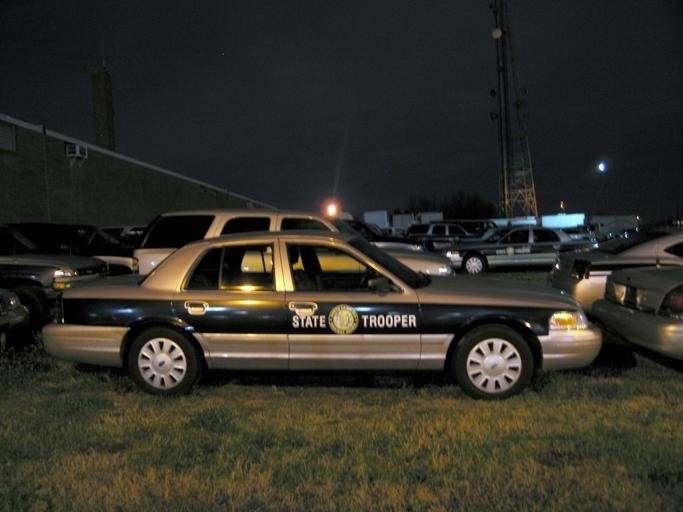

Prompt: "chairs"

[287,244,318,290]
